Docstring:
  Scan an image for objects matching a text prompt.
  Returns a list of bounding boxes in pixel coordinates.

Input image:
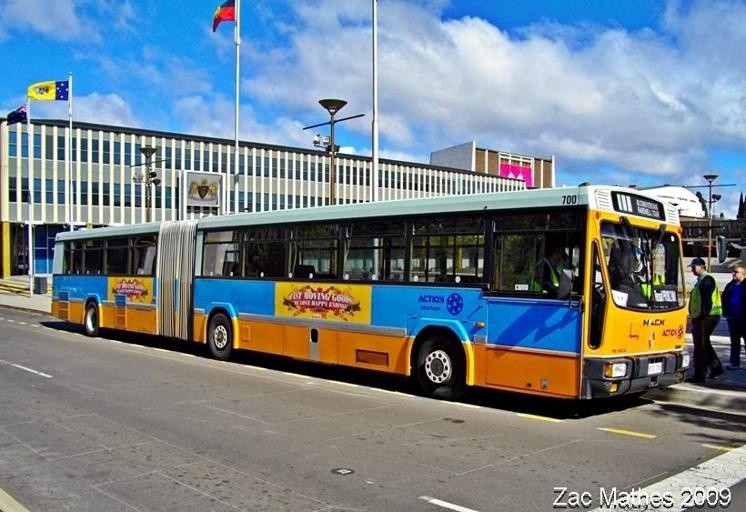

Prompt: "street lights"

[695,174,724,277]
[301,99,366,205]
[128,148,166,224]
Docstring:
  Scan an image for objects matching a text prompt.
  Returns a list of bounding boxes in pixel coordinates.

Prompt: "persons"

[721,263,746,370]
[683,258,725,383]
[530,246,567,292]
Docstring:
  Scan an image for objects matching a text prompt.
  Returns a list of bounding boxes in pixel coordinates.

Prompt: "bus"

[47,183,728,406]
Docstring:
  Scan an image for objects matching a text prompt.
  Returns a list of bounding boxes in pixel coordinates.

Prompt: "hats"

[687,258,705,266]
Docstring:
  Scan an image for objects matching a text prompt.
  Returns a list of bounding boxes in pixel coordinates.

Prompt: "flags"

[6,103,28,126]
[212,0,236,32]
[27,81,69,101]
